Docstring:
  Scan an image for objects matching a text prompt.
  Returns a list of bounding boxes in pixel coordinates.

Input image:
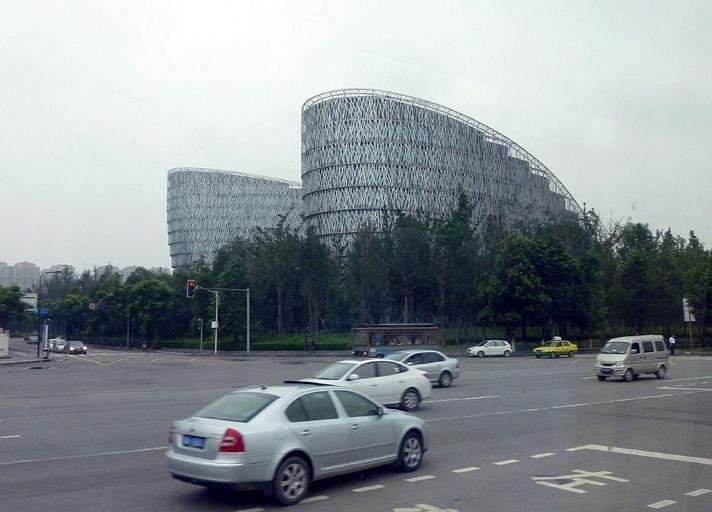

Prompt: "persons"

[668,334,675,355]
[307,334,315,354]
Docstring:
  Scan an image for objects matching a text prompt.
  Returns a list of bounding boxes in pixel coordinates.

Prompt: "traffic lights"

[186,279,196,298]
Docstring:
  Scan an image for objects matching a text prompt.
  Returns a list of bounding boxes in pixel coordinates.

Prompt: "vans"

[594,334,670,383]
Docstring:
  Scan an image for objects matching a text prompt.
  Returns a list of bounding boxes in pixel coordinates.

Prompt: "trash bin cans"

[142,341,148,349]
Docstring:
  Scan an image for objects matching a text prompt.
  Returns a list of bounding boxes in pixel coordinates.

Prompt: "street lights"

[37,269,63,358]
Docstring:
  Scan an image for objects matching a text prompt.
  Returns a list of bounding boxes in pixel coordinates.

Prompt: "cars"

[299,348,462,412]
[164,379,431,504]
[24,335,88,355]
[532,336,578,358]
[465,339,514,358]
